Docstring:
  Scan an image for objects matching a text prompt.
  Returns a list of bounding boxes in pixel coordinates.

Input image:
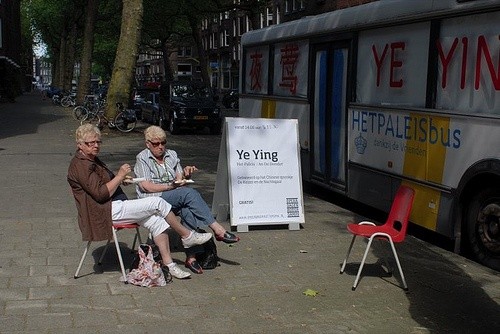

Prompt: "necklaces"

[154,161,170,183]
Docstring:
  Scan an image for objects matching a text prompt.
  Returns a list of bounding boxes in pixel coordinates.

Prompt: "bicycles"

[46,86,137,133]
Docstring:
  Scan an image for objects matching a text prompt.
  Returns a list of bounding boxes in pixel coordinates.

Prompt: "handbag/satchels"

[129,244,173,284]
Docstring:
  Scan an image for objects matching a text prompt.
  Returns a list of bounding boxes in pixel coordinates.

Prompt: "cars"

[133,88,146,108]
[141,91,160,125]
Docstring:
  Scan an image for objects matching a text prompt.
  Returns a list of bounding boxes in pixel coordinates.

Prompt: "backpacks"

[186,227,218,270]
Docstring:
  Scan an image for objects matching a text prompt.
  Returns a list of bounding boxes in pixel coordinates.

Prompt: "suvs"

[159,80,222,136]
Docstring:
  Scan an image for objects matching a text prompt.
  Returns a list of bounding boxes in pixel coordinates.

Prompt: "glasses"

[81,140,102,147]
[147,140,166,147]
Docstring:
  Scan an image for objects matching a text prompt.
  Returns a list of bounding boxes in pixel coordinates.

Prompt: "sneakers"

[166,262,191,278]
[181,230,212,248]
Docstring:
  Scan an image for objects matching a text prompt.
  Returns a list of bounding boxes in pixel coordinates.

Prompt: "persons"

[134,125,240,275]
[68,123,213,279]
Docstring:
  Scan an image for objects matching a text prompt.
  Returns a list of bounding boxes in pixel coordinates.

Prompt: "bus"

[237,0,500,279]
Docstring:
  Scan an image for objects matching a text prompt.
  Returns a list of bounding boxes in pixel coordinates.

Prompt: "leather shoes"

[214,230,240,243]
[185,258,203,274]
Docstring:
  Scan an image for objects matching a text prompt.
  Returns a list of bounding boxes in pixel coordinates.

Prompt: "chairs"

[74,223,142,284]
[339,185,416,294]
